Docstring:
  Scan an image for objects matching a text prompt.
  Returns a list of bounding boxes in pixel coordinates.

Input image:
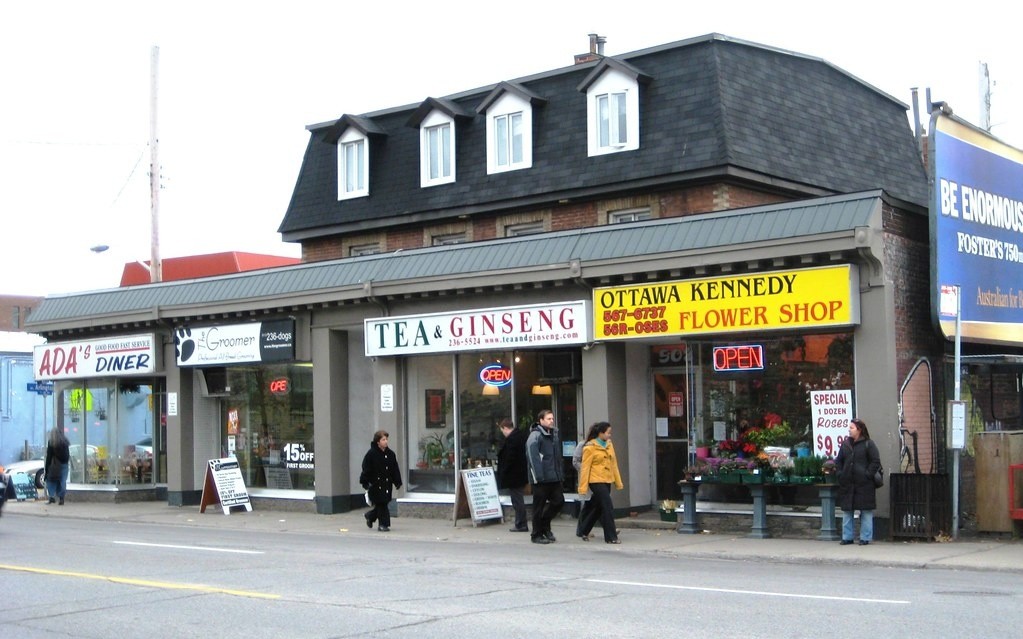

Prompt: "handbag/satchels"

[874,464,884,488]
[47,456,62,483]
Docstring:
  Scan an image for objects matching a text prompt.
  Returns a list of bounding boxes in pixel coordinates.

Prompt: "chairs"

[69,451,152,485]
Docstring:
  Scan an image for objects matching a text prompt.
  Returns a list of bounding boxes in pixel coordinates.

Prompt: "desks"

[253,464,278,487]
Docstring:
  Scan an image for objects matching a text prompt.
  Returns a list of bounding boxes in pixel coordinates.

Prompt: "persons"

[497,418,528,532]
[836,419,880,545]
[360,431,402,531]
[44,427,69,505]
[525,409,565,543]
[572,422,623,544]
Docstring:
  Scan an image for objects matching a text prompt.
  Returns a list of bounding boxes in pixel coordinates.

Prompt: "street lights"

[91,243,164,282]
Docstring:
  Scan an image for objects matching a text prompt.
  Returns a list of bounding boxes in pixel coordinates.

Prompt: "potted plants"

[788,454,822,484]
[694,439,708,458]
[659,499,677,522]
[797,442,810,459]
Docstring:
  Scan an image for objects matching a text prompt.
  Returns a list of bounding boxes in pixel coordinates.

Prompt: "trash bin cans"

[888,472,951,543]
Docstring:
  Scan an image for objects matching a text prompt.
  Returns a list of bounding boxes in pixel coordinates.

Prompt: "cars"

[123,437,154,471]
[1,443,103,488]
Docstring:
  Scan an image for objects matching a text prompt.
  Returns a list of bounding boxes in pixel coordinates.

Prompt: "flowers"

[682,412,796,472]
[822,460,835,474]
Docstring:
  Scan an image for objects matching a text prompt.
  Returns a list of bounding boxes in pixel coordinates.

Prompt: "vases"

[774,471,788,483]
[825,474,835,484]
[685,470,763,485]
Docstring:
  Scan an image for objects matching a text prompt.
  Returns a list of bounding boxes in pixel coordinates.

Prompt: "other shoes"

[510,527,528,532]
[59,499,64,504]
[531,536,550,544]
[365,513,373,528]
[49,498,55,504]
[858,540,869,545]
[544,531,555,541]
[589,533,594,537]
[607,539,621,544]
[616,529,621,534]
[378,526,390,532]
[840,540,854,545]
[577,534,589,541]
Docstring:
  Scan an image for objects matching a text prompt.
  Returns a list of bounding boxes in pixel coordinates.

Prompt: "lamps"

[477,350,521,365]
[481,384,499,396]
[531,385,551,395]
